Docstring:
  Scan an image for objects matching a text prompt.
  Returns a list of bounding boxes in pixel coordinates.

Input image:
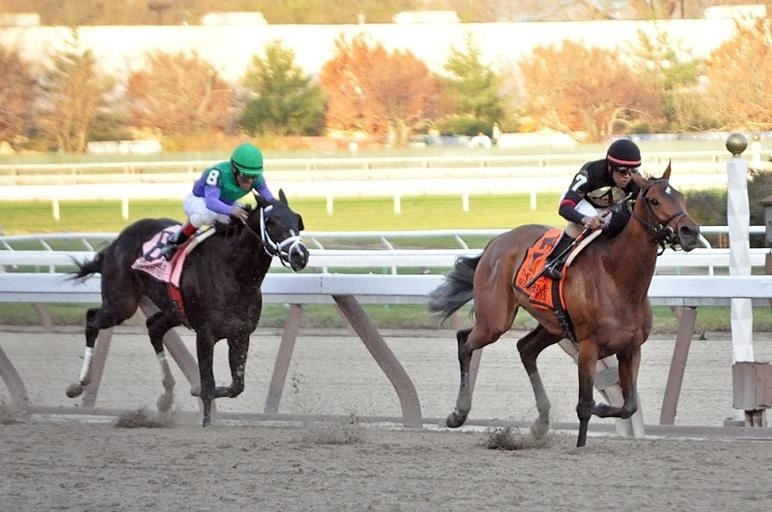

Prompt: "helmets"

[231,143,264,176]
[605,139,642,170]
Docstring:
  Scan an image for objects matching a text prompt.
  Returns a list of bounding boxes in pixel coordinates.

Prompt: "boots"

[161,229,189,262]
[544,230,576,281]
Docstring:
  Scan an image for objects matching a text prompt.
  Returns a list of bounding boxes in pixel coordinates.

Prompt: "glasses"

[606,160,638,175]
[231,161,258,181]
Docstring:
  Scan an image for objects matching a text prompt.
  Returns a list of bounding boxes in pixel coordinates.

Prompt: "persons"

[160,142,273,261]
[542,139,642,280]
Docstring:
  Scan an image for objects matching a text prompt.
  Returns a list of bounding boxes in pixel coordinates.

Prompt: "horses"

[60,187,310,426]
[423,156,700,446]
